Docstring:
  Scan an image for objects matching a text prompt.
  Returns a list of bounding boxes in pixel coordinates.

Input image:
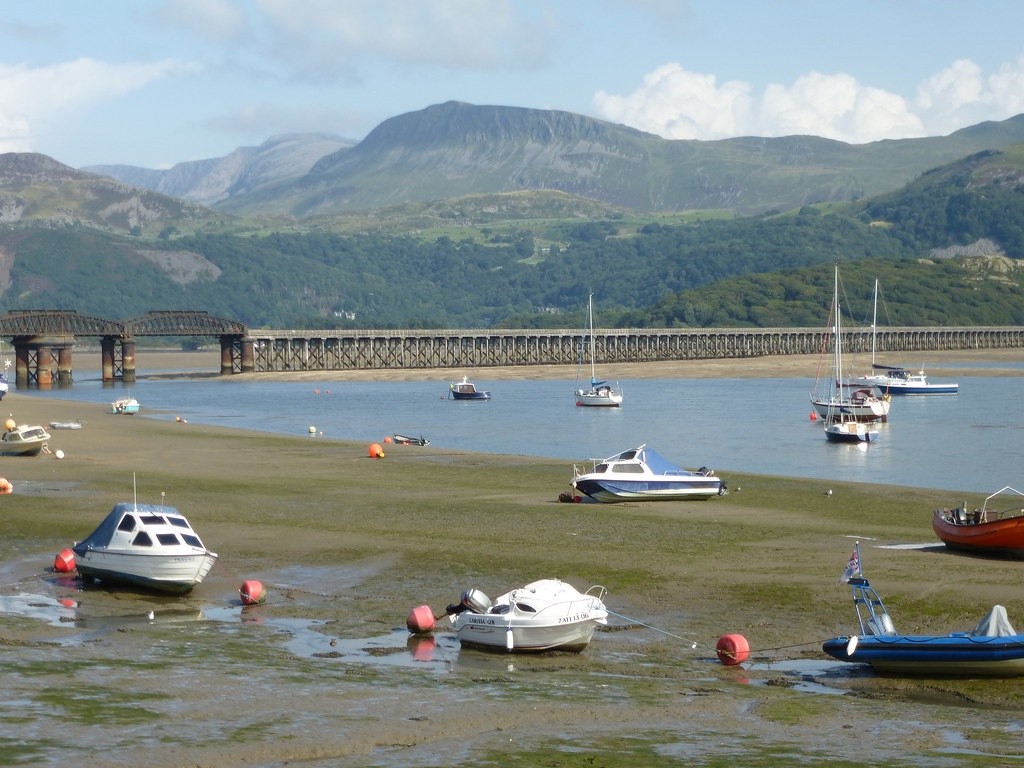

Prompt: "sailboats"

[822,302,880,443]
[573,291,623,407]
[809,263,891,421]
[834,279,928,387]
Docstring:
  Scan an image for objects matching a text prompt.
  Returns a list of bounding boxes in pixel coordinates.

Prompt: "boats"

[445,576,610,654]
[111,397,140,415]
[72,471,218,597]
[393,433,430,446]
[873,370,961,397]
[569,443,728,503]
[0,419,51,457]
[0,373,8,403]
[822,539,1024,680]
[931,484,1024,559]
[450,374,491,400]
